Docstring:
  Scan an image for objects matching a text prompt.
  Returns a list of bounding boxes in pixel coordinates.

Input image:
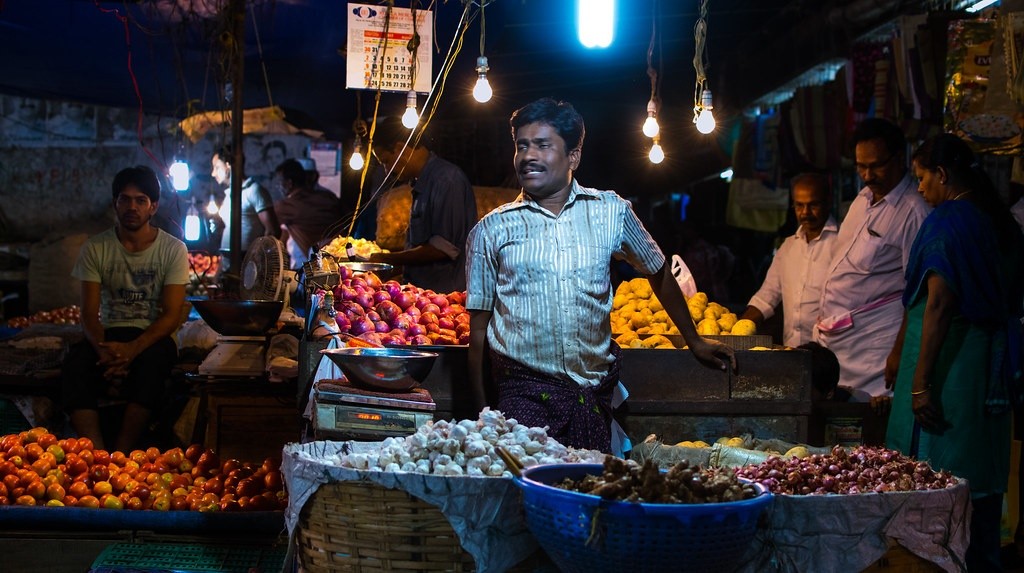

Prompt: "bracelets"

[911,390,927,394]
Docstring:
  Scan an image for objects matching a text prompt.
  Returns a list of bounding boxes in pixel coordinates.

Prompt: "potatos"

[608,278,765,350]
[676,435,809,459]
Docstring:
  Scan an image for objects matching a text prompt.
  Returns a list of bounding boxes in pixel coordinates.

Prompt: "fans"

[239,235,304,323]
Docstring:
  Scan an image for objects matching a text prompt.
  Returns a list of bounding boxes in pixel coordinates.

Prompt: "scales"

[309,379,436,438]
[184,296,285,375]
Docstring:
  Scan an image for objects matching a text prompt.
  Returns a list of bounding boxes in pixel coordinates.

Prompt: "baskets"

[290,479,552,573]
[515,463,775,573]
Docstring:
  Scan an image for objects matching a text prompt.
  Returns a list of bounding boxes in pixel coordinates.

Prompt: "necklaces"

[954,190,971,200]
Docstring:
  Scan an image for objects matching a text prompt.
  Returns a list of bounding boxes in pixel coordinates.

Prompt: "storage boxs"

[611,325,811,447]
[89,540,294,573]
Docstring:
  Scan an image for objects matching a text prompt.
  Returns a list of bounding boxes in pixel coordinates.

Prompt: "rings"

[920,415,924,416]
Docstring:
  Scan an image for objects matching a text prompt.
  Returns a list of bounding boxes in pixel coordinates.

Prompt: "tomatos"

[0,428,279,509]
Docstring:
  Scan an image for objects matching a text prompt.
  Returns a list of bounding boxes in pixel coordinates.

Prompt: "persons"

[211,144,282,268]
[273,158,351,268]
[742,173,840,348]
[466,98,737,451]
[369,116,478,293]
[812,119,935,446]
[61,165,192,453]
[886,134,1024,573]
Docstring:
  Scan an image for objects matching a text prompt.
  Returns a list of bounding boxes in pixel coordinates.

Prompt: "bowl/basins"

[341,261,392,274]
[318,346,440,392]
[191,299,285,335]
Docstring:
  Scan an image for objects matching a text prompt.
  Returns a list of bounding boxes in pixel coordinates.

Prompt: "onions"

[8,304,80,329]
[187,252,221,275]
[321,234,391,258]
[738,445,956,495]
[331,264,471,346]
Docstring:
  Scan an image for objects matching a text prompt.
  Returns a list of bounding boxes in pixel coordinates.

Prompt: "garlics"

[321,408,601,479]
